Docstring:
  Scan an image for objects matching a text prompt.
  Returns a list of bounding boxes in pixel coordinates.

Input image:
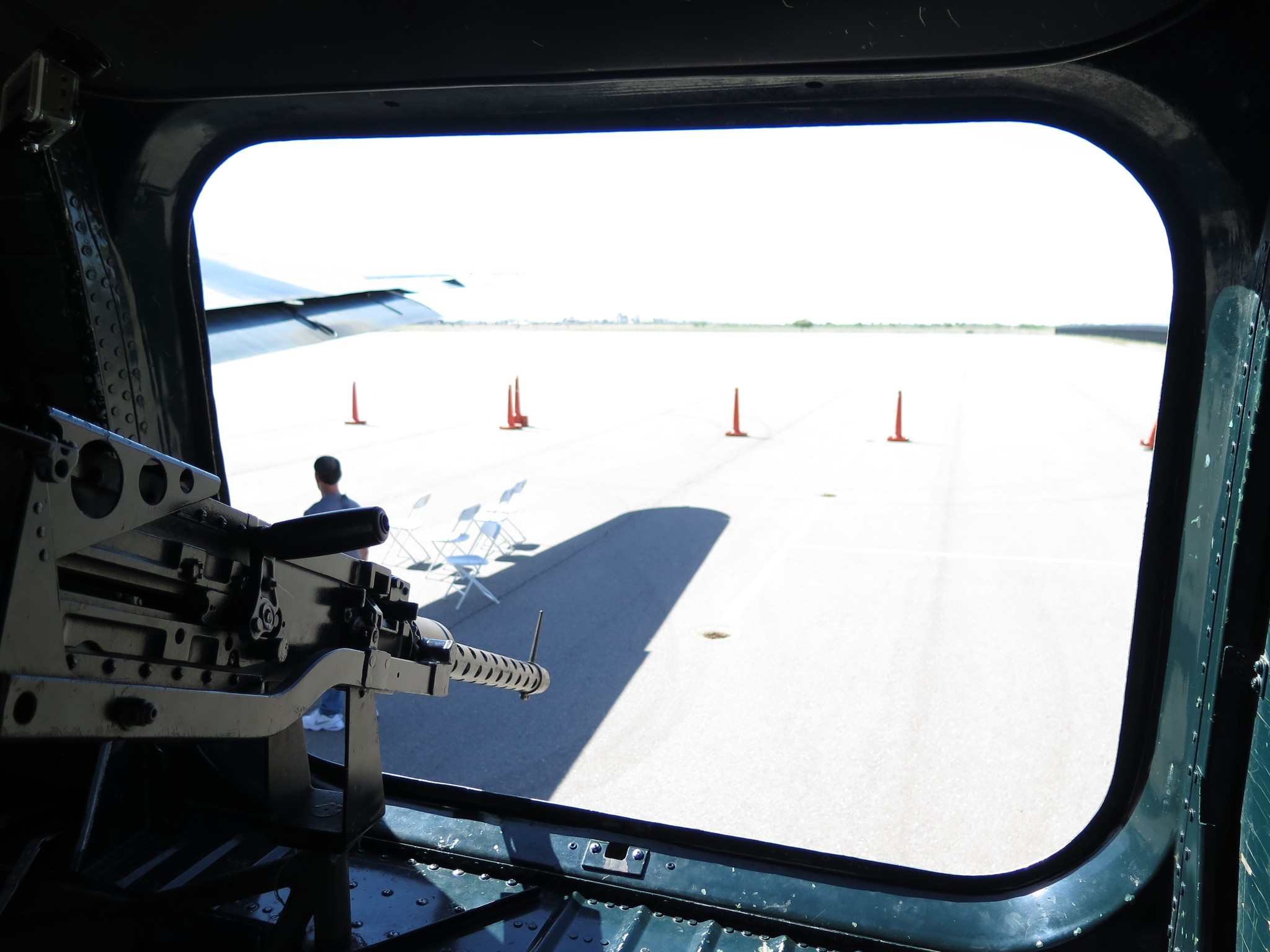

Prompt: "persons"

[302,455,370,732]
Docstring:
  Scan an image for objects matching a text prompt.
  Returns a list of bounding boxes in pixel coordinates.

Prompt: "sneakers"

[302,709,345,732]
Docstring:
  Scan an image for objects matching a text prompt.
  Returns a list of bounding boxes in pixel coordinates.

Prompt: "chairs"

[382,495,431,567]
[469,479,527,557]
[425,503,482,579]
[443,522,501,612]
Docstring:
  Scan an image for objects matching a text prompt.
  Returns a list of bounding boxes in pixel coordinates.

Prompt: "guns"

[1,402,555,849]
